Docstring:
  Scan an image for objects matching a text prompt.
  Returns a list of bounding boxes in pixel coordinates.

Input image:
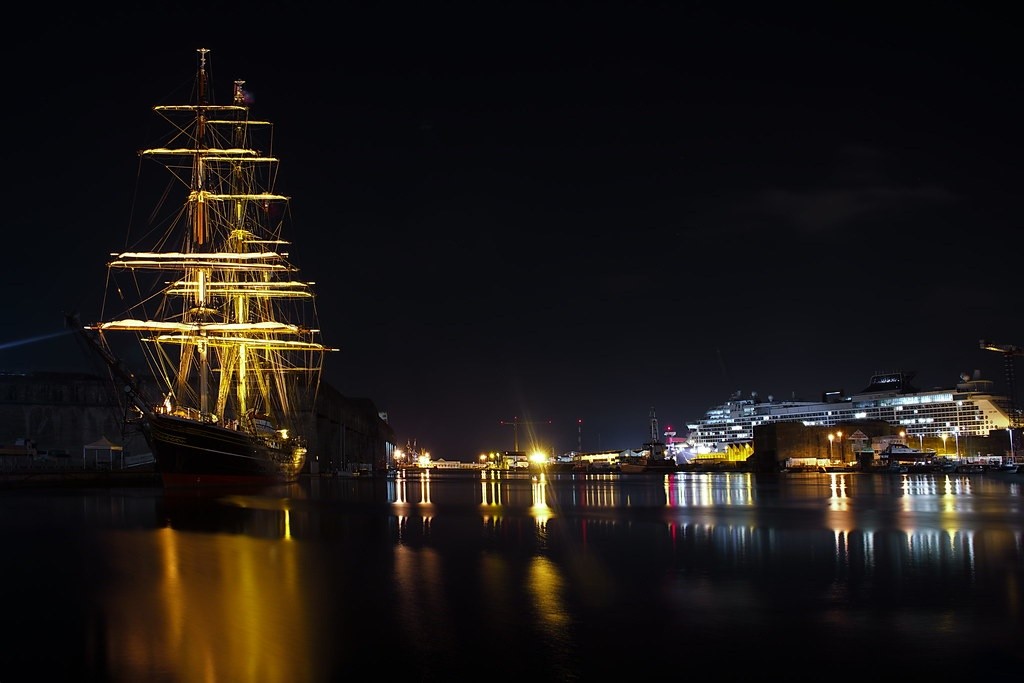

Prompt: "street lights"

[828,433,836,462]
[836,429,844,464]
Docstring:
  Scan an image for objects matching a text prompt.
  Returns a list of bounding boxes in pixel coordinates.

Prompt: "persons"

[164,391,177,416]
[235,419,290,450]
[877,456,1012,468]
[575,460,622,472]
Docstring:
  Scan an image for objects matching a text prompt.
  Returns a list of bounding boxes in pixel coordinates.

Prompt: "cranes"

[980,338,1024,458]
[500,416,552,453]
[575,417,584,451]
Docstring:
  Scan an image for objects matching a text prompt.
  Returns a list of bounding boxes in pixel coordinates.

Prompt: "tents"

[83,435,123,471]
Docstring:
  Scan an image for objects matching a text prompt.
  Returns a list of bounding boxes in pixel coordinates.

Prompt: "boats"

[875,443,1019,474]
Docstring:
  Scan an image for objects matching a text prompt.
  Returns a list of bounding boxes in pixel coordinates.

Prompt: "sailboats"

[58,43,342,480]
